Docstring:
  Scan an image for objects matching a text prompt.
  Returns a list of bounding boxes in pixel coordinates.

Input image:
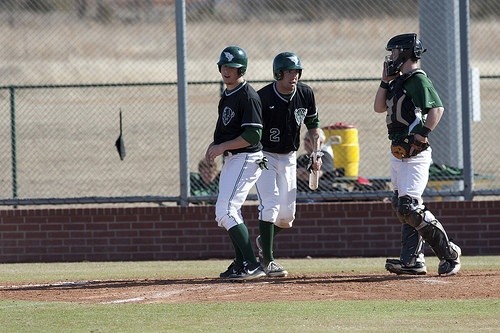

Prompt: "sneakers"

[438,242,461,275]
[220,260,266,280]
[385,259,427,275]
[256,234,273,261]
[259,259,288,276]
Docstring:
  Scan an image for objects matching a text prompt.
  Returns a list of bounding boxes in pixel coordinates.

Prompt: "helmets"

[386,32,427,75]
[273,50,304,80]
[217,45,247,75]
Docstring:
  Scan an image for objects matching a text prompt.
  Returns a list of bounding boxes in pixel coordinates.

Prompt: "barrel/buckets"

[322,123,360,178]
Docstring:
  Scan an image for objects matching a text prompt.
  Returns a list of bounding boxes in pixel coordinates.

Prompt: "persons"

[295,128,335,202]
[189,159,221,204]
[374,33,463,276]
[256,52,324,276]
[205,46,263,279]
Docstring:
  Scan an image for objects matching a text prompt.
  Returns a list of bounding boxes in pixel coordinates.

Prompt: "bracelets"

[416,126,431,137]
